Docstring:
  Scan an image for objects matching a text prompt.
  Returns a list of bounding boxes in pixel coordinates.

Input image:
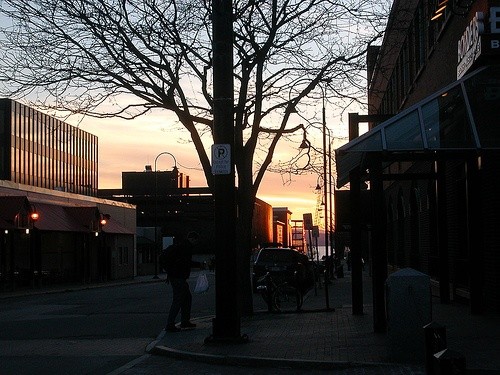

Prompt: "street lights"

[283,77,330,310]
[299,121,334,280]
[152,151,179,279]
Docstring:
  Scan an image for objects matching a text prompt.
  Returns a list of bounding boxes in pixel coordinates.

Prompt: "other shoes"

[166,326,181,332]
[180,321,196,328]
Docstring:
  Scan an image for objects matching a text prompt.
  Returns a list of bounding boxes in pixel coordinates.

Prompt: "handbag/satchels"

[194,269,209,296]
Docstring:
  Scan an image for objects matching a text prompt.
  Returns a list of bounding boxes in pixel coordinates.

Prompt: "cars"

[254,245,326,294]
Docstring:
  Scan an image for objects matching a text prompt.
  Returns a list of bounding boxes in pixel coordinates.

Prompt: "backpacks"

[163,243,177,284]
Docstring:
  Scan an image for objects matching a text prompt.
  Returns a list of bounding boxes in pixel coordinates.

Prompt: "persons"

[167,231,200,332]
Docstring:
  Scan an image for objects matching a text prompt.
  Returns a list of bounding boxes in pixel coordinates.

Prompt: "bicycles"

[252,268,302,313]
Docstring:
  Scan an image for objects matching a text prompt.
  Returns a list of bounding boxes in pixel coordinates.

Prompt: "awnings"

[0,196,136,235]
[335,64,500,189]
